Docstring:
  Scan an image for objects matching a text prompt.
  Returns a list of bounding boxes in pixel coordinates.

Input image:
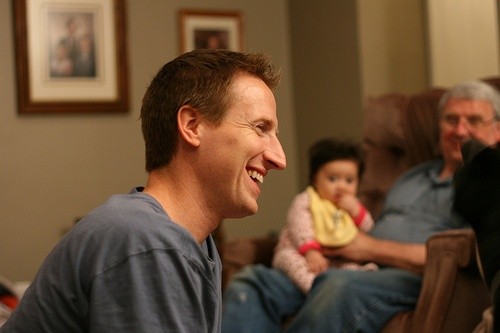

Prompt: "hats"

[309,139,366,182]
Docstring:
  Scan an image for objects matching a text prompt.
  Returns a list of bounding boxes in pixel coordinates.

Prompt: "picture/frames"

[13,0,131,116]
[177,7,246,56]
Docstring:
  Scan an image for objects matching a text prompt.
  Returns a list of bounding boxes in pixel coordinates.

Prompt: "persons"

[49,18,94,79]
[222,81,500,333]
[272,138,378,292]
[0,48,286,333]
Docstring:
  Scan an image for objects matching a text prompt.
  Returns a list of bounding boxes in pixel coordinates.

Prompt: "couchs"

[221,77,500,333]
